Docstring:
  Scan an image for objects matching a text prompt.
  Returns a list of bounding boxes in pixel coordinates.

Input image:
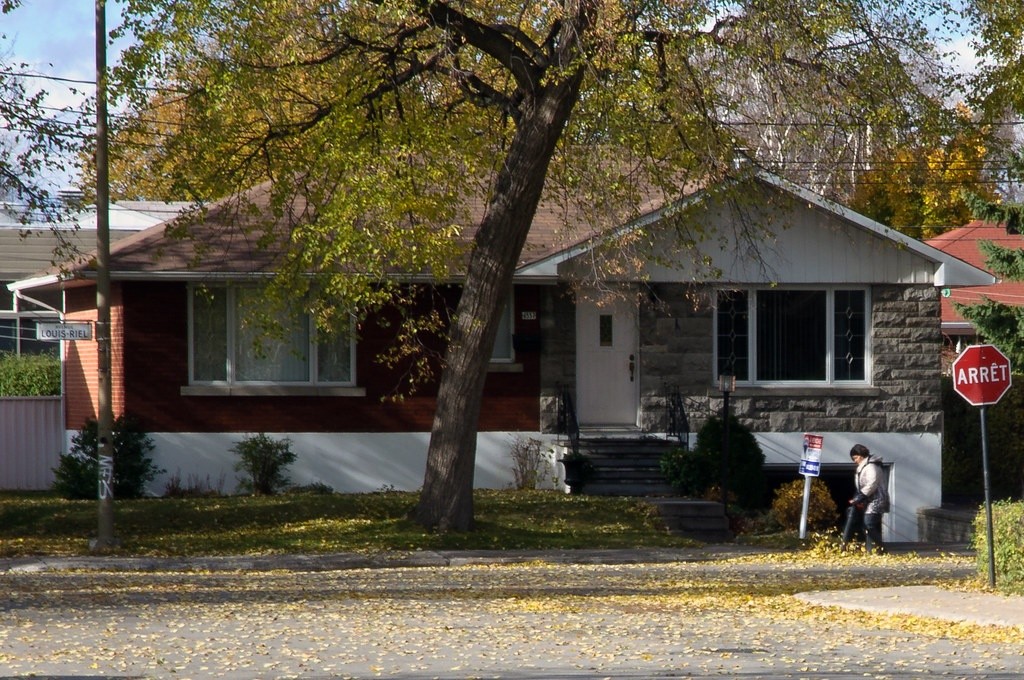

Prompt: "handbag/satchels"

[849,492,867,510]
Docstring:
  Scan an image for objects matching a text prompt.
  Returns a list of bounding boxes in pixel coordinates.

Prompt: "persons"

[841,444,890,557]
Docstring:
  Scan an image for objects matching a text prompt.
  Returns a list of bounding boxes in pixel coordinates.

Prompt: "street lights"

[719,352,736,514]
